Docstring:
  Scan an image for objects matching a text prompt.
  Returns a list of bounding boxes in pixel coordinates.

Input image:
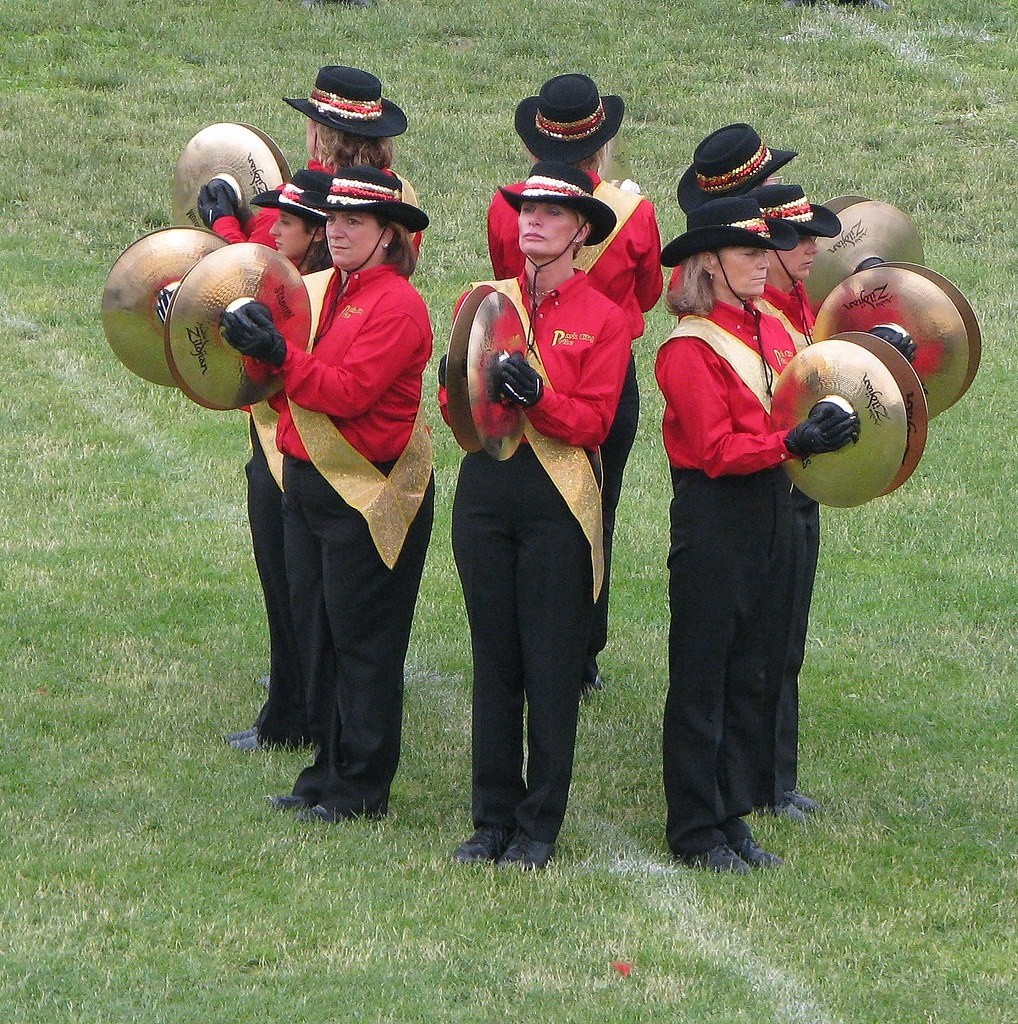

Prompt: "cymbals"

[772,195,981,509]
[98,119,313,411]
[613,175,645,198]
[445,283,527,462]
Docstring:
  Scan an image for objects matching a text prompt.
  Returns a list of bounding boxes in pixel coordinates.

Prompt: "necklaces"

[523,286,554,298]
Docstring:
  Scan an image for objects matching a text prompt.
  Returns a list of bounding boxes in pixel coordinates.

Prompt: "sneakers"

[458,824,557,872]
[680,840,789,876]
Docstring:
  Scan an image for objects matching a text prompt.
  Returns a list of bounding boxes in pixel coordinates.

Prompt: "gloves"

[883,329,922,369]
[437,355,447,391]
[782,402,866,457]
[218,305,287,368]
[193,182,240,230]
[499,354,547,407]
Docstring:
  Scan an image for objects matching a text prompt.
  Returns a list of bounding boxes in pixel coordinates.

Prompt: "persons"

[487,75,663,703]
[437,159,631,872]
[654,125,917,876]
[153,65,435,826]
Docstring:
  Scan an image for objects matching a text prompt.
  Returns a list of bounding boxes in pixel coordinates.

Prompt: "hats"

[496,162,618,247]
[248,166,429,234]
[677,124,802,215]
[731,185,842,238]
[510,73,626,167]
[284,65,411,139]
[659,197,801,273]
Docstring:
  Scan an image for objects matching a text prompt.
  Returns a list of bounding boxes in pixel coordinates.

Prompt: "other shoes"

[274,789,369,822]
[776,794,820,822]
[224,725,272,751]
